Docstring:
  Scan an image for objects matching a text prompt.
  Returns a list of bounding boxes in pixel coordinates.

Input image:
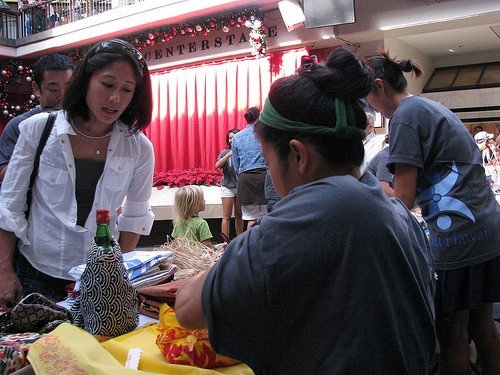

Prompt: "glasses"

[100,40,145,68]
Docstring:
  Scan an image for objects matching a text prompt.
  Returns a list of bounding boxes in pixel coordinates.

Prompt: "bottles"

[94,208,114,248]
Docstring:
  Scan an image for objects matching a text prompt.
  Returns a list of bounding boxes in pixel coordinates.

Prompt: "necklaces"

[71,119,123,155]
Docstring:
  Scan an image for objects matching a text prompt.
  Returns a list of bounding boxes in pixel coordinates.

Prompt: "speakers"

[278,0,305,32]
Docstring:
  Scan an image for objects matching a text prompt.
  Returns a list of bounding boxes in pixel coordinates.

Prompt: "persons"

[171,185,213,246]
[175,46,441,375]
[0,0,102,35]
[215,106,409,239]
[0,53,77,182]
[0,39,155,308]
[365,50,500,375]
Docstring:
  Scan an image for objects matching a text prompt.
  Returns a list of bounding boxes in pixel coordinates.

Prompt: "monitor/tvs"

[304,0,356,30]
